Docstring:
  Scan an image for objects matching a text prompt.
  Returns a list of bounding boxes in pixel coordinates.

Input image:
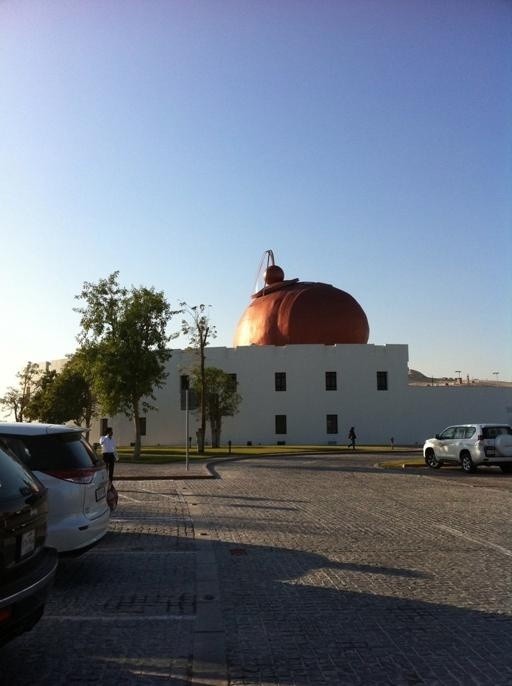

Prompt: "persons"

[348,427,356,449]
[100,427,120,483]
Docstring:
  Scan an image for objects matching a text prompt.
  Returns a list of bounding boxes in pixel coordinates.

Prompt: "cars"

[0,421,118,649]
[423,423,512,473]
